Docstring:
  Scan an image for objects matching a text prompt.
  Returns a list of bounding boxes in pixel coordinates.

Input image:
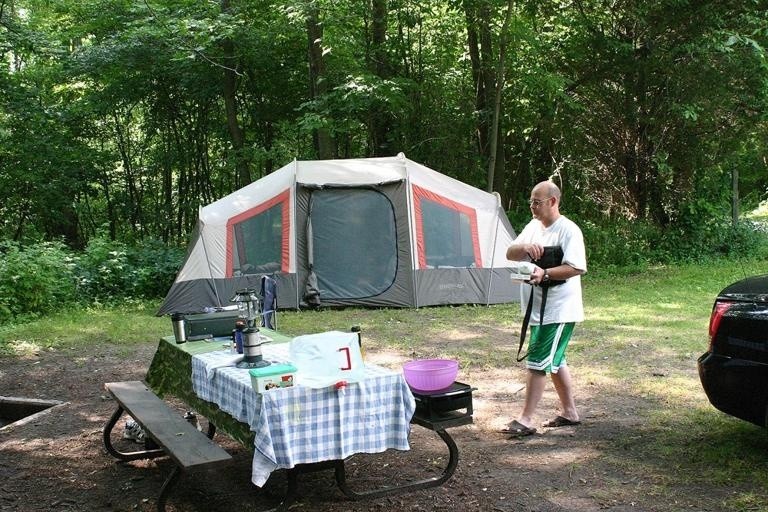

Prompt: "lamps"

[228,289,261,334]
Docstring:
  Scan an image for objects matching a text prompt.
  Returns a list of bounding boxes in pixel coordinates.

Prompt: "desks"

[160,326,406,503]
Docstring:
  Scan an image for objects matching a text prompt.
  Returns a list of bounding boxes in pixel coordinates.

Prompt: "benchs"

[410,411,474,492]
[101,380,233,512]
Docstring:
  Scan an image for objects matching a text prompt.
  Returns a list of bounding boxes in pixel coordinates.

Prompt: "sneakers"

[495,420,537,434]
[541,415,581,427]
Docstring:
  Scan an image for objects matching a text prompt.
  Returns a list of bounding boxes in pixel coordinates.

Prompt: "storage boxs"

[185,311,239,338]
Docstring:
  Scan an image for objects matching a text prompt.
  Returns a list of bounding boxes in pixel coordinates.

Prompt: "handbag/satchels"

[526,246,567,289]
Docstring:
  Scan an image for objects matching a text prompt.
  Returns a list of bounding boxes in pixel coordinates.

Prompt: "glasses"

[527,196,555,206]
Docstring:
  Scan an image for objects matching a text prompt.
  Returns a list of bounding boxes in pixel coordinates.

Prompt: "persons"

[494,181,588,437]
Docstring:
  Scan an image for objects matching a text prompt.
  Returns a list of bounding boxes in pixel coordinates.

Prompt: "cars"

[696,274,768,430]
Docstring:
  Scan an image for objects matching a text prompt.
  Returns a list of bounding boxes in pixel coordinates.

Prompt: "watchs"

[542,268,549,281]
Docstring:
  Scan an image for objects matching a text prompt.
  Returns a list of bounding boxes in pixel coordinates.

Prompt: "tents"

[153,150,531,318]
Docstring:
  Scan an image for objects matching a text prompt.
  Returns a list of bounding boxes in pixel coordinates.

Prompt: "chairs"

[258,274,279,330]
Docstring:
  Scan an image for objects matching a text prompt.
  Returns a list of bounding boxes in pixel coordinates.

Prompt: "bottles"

[235,321,248,354]
[171,312,187,344]
